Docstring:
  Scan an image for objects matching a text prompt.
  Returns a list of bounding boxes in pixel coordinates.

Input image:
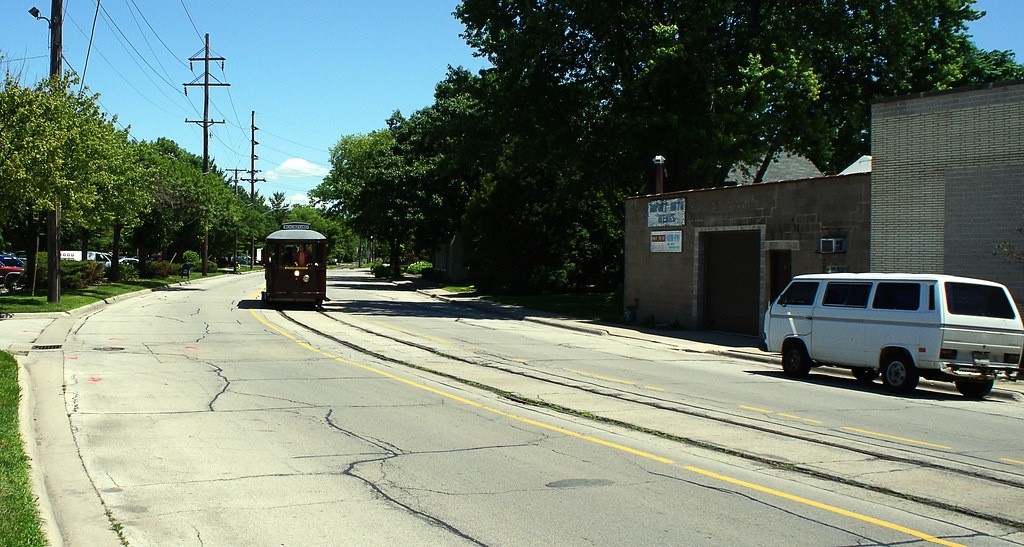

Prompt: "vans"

[760,270,1024,398]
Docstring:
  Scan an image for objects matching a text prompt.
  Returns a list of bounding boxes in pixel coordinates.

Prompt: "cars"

[88,251,140,270]
[0,250,28,292]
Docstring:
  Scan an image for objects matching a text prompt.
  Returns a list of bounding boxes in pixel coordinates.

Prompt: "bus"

[262,221,329,310]
